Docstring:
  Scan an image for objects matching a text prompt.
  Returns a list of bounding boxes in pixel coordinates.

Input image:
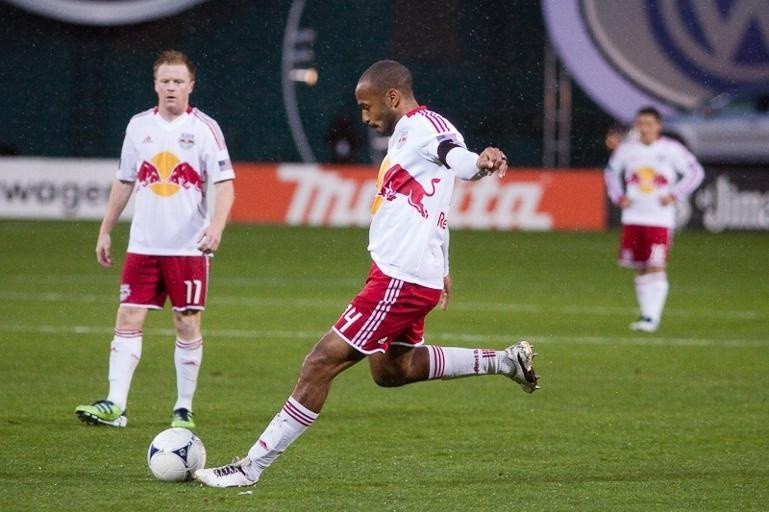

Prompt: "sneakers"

[629,316,659,333]
[170,407,196,428]
[504,339,541,394]
[74,399,128,427]
[192,456,259,488]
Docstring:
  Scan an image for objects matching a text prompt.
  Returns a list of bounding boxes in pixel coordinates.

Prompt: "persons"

[194,61,540,489]
[74,50,235,429]
[602,106,705,333]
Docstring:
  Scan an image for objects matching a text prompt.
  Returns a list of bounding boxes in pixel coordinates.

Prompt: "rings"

[502,155,508,160]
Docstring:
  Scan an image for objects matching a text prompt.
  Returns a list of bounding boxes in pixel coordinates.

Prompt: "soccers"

[146,427,206,484]
